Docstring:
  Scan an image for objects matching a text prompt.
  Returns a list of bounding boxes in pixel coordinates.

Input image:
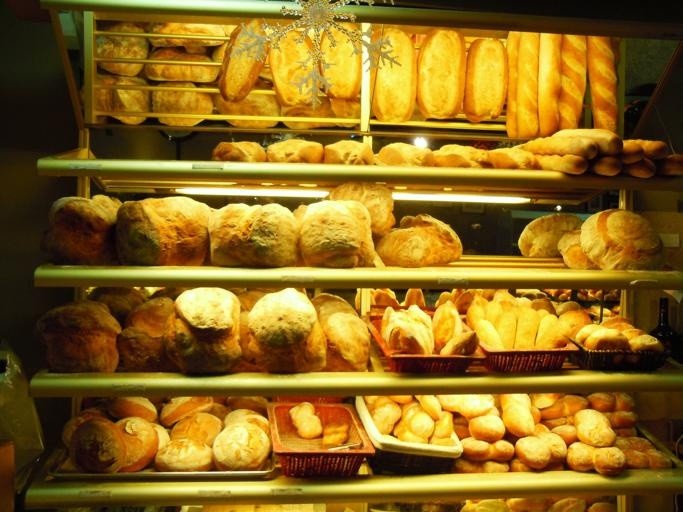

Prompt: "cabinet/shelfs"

[26,1,683,512]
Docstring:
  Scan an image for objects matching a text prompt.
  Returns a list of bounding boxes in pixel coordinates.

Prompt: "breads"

[575,325,606,344]
[108,396,158,420]
[377,142,434,167]
[502,403,534,437]
[552,497,585,512]
[93,74,149,125]
[493,440,514,460]
[149,22,225,46]
[212,423,270,471]
[153,82,213,127]
[470,414,506,442]
[373,28,414,123]
[419,395,440,421]
[398,432,428,445]
[268,140,323,163]
[518,212,583,257]
[475,321,505,351]
[515,436,551,469]
[112,417,159,471]
[154,438,212,471]
[533,299,557,317]
[594,158,622,177]
[389,395,412,404]
[593,446,625,475]
[508,31,538,139]
[623,329,649,341]
[532,153,587,177]
[374,405,400,434]
[221,22,271,104]
[434,411,453,438]
[612,411,637,425]
[584,329,630,351]
[627,436,653,451]
[269,30,320,105]
[577,422,615,448]
[330,181,395,238]
[320,22,360,100]
[558,35,587,127]
[615,392,633,411]
[538,32,560,134]
[160,396,213,426]
[383,306,432,355]
[575,409,611,426]
[579,209,667,271]
[589,393,613,412]
[487,148,536,169]
[438,394,494,417]
[587,35,619,131]
[509,497,549,510]
[36,301,121,373]
[548,289,620,301]
[647,447,672,468]
[410,413,434,437]
[536,315,583,349]
[556,301,581,318]
[559,310,592,340]
[433,144,478,169]
[461,437,491,462]
[373,292,399,306]
[169,412,223,443]
[521,136,597,158]
[163,287,242,375]
[378,213,462,267]
[620,142,644,164]
[312,293,371,373]
[289,402,315,419]
[405,288,425,306]
[225,408,269,434]
[295,200,375,268]
[660,154,683,176]
[44,194,120,264]
[146,48,219,82]
[616,427,638,438]
[496,312,517,351]
[541,393,588,419]
[465,37,508,121]
[457,290,533,321]
[440,332,480,356]
[430,437,454,448]
[323,423,349,445]
[558,230,598,271]
[248,288,326,374]
[552,424,577,445]
[415,30,466,120]
[623,139,668,158]
[208,203,295,267]
[69,418,126,475]
[542,433,568,460]
[297,414,323,439]
[623,449,648,468]
[625,159,656,179]
[630,334,664,352]
[567,442,596,471]
[96,24,148,77]
[214,94,281,128]
[213,140,266,164]
[555,128,622,155]
[117,197,209,265]
[225,396,269,414]
[118,294,176,372]
[517,309,538,349]
[484,460,508,472]
[434,300,462,353]
[324,138,375,166]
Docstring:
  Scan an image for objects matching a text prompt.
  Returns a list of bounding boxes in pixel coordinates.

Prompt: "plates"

[47,441,276,481]
